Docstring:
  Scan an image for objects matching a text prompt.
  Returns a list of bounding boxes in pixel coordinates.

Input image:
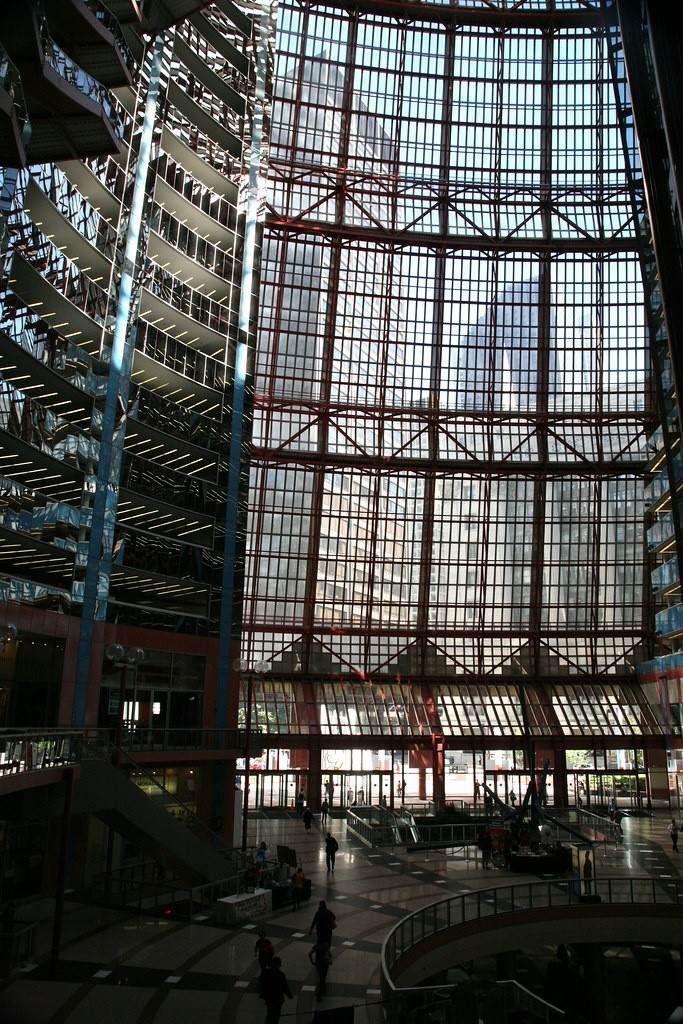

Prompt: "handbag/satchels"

[324,946,333,967]
[327,911,338,930]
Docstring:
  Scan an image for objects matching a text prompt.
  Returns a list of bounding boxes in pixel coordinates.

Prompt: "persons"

[397,779,407,798]
[646,800,655,817]
[508,789,517,807]
[323,780,330,798]
[307,933,332,1001]
[479,831,493,870]
[321,799,329,825]
[610,799,617,821]
[325,832,339,871]
[256,957,294,1024]
[303,807,315,833]
[382,795,387,806]
[260,842,267,851]
[613,807,624,835]
[579,797,583,809]
[669,819,680,853]
[253,931,271,974]
[297,789,305,814]
[475,779,482,801]
[290,866,305,912]
[309,899,337,948]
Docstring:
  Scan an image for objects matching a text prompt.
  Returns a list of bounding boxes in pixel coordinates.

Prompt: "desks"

[510,856,573,874]
[272,879,294,908]
[216,889,273,928]
